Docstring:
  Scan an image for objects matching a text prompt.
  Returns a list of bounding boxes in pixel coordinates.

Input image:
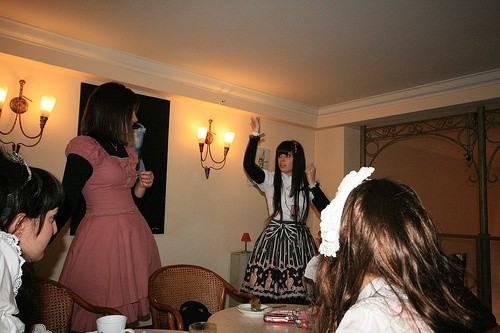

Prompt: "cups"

[95,315,135,333]
[189,322,217,333]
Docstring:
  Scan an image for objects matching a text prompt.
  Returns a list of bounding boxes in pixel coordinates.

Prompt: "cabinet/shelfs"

[229,253,251,308]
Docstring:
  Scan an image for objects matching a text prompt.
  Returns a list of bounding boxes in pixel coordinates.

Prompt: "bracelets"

[251,131,265,137]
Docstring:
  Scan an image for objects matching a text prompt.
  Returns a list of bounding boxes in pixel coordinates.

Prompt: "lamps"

[240,233,251,253]
[0,80,56,154]
[198,119,235,179]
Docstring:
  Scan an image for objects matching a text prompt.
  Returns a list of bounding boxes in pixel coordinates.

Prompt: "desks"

[208,304,315,333]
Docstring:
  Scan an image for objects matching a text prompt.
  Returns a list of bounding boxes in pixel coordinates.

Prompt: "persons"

[243,117,330,306]
[61,83,161,332]
[308,167,500,333]
[0,143,63,333]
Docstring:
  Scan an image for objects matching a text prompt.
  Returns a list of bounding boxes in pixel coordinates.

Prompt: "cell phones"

[263,312,295,324]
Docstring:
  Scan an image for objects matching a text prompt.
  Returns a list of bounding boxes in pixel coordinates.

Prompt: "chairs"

[11,278,128,333]
[148,264,259,331]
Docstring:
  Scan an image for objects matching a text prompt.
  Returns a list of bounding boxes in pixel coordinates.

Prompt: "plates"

[237,303,272,315]
